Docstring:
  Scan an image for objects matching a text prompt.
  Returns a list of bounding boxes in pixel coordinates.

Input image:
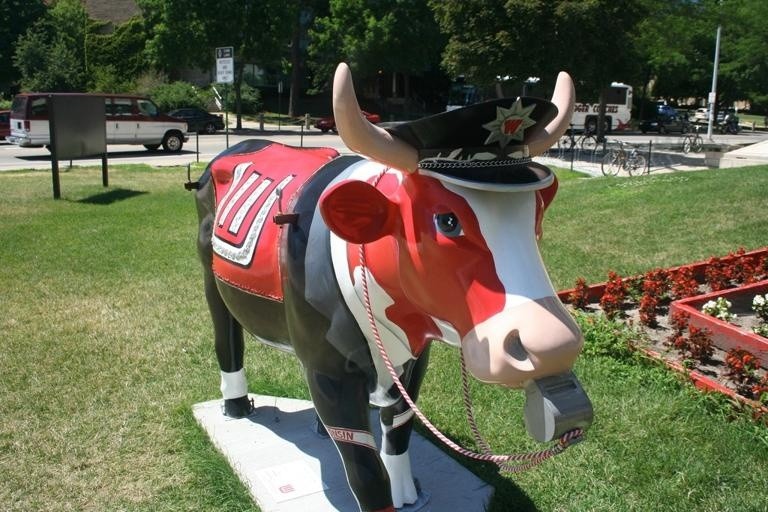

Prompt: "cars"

[312,111,381,134]
[0,109,12,140]
[638,104,740,134]
[167,107,226,135]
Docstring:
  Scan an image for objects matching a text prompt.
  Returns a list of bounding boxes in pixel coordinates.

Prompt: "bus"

[446,75,634,134]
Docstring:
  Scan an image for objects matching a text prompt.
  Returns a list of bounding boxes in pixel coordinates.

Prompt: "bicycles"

[558,122,598,155]
[681,123,703,154]
[600,140,647,177]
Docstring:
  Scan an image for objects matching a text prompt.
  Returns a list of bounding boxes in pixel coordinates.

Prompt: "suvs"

[5,92,189,159]
